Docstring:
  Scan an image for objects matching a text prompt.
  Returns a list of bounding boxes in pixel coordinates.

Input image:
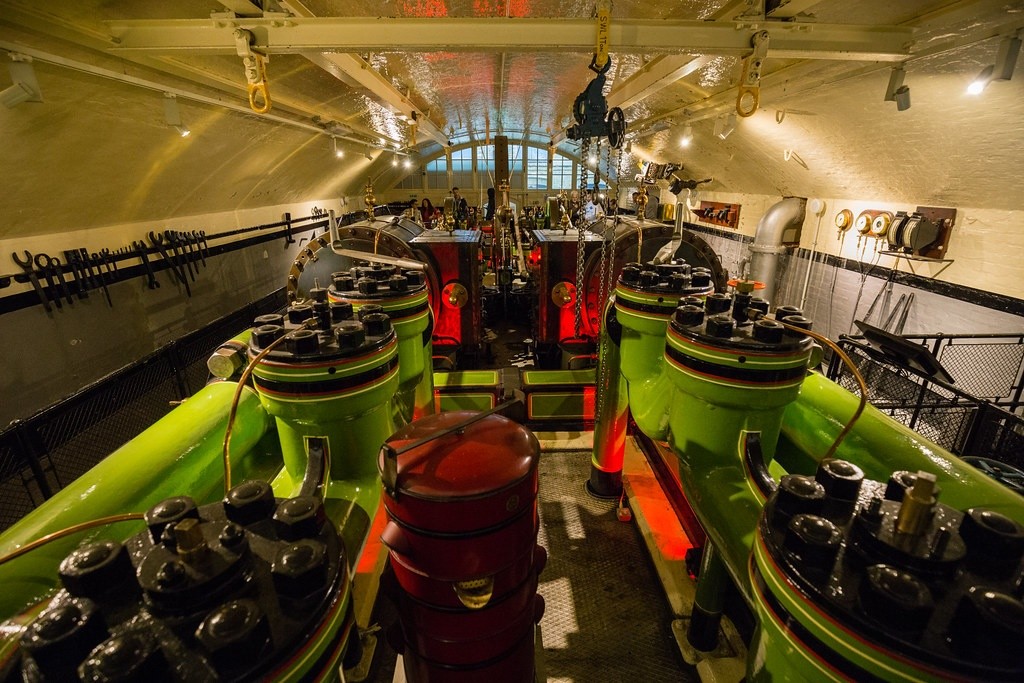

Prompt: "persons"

[633,190,658,218]
[568,189,624,227]
[400,199,427,229]
[452,187,470,229]
[421,198,444,229]
